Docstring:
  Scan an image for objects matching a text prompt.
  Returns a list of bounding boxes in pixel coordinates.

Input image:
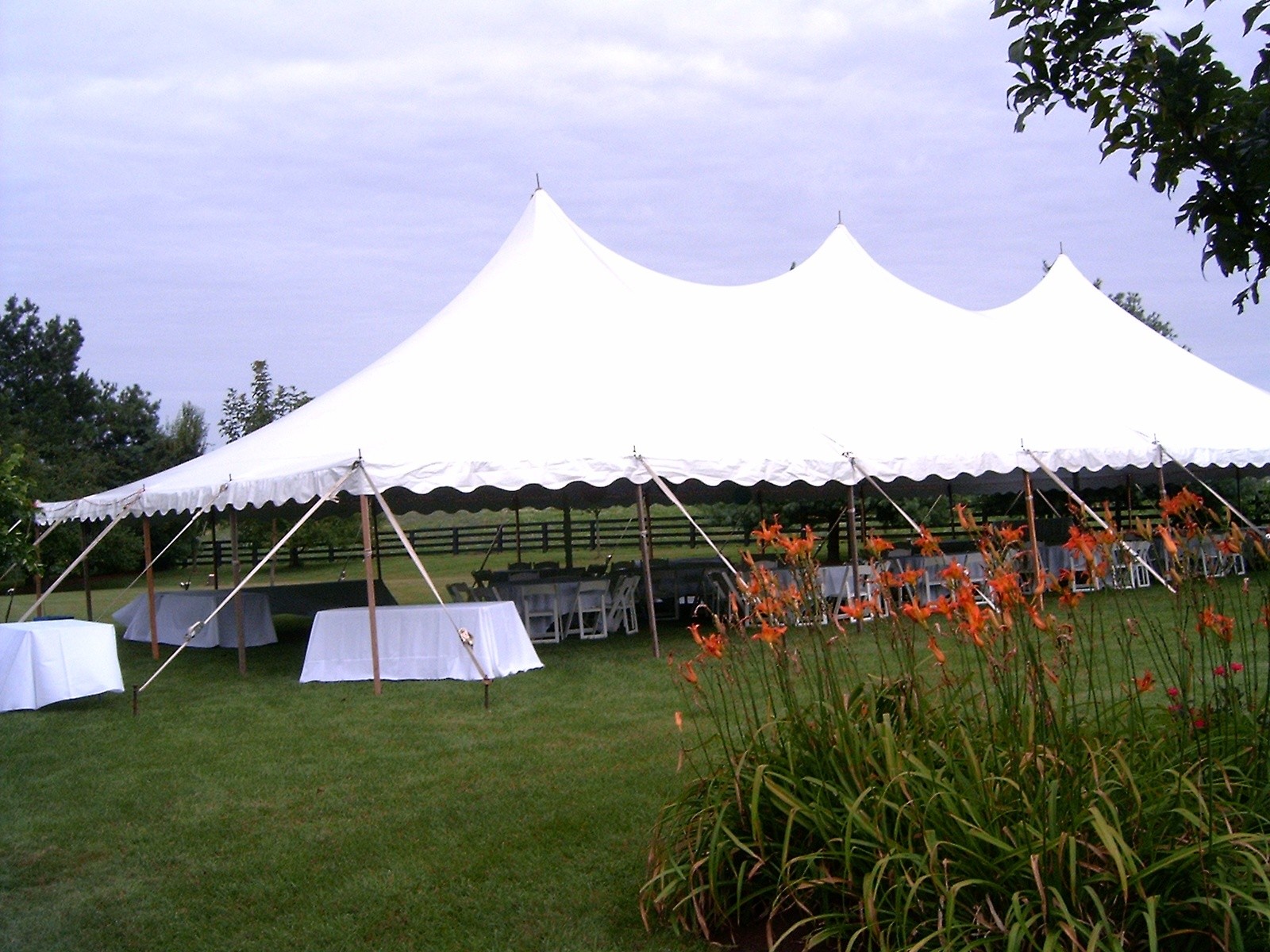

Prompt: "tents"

[25,174,1270,662]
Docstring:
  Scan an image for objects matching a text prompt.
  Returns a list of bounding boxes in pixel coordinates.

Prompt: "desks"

[607,555,731,619]
[743,561,874,628]
[1030,536,1154,596]
[492,569,614,637]
[1155,526,1245,575]
[0,614,129,719]
[297,595,548,689]
[114,589,280,650]
[884,549,996,615]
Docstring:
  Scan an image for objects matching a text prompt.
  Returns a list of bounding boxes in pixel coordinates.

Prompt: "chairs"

[521,582,564,645]
[565,578,611,641]
[789,567,827,628]
[607,574,642,637]
[751,559,781,571]
[917,555,958,613]
[959,556,1000,610]
[648,569,681,623]
[687,567,745,629]
[470,586,503,602]
[474,568,493,587]
[610,559,637,572]
[533,559,562,569]
[832,564,874,625]
[640,556,670,569]
[1021,539,1045,551]
[1068,532,1247,598]
[888,546,915,558]
[447,582,473,603]
[509,560,531,570]
